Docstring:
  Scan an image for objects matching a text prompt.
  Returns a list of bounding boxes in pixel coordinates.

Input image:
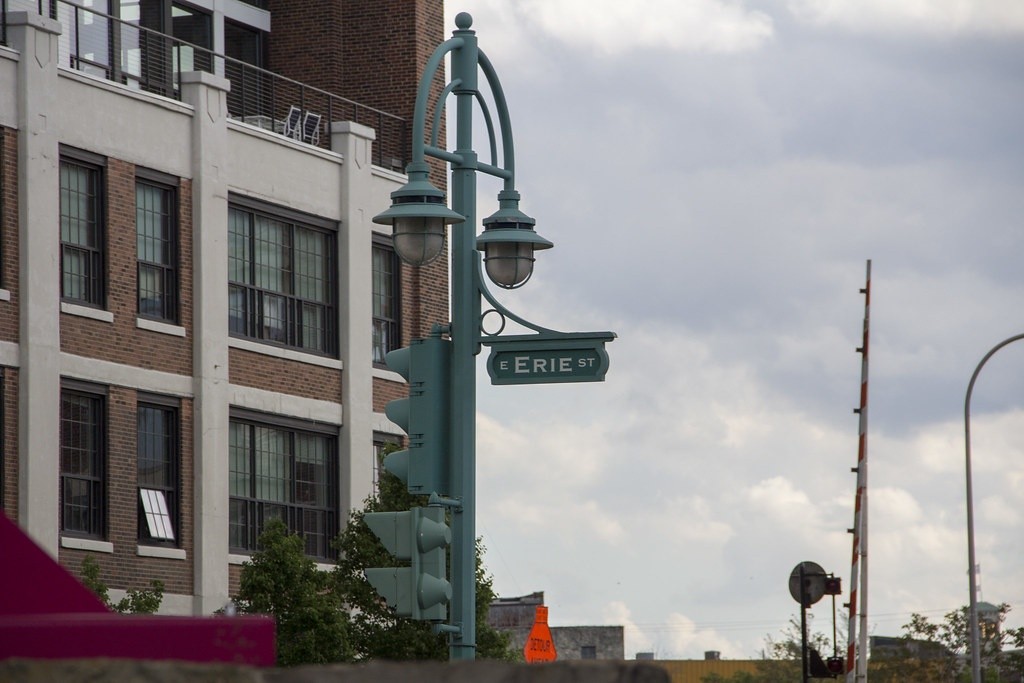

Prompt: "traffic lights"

[380,336,450,496]
[363,502,450,627]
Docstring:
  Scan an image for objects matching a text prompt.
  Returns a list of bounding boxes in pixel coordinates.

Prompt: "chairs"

[280,104,323,146]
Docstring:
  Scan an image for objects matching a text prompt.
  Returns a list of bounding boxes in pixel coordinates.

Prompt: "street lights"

[375,10,554,656]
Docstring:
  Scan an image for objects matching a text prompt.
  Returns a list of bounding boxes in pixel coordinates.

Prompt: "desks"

[231,115,287,132]
